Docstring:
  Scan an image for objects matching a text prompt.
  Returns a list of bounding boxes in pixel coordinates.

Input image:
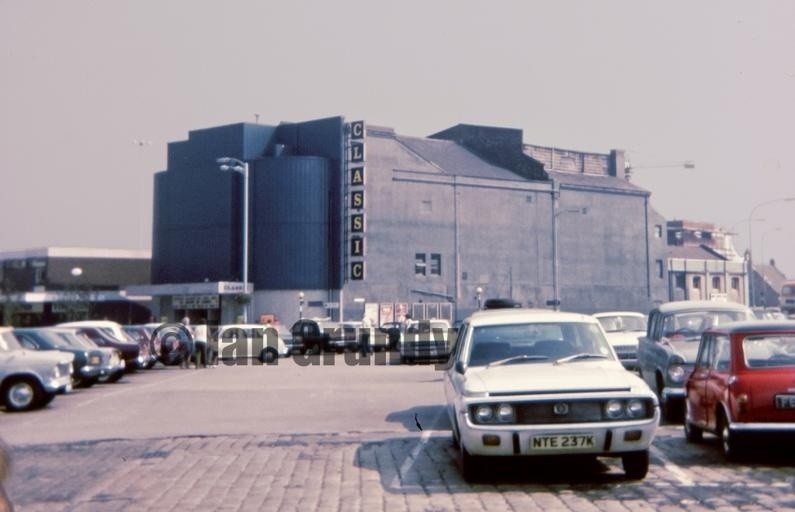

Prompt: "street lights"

[475,285,483,311]
[746,195,795,308]
[630,160,696,172]
[297,291,305,322]
[724,216,767,296]
[551,206,590,310]
[759,224,783,296]
[215,157,250,324]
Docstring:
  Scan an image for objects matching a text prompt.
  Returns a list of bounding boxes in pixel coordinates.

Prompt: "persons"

[176,316,193,369]
[404,314,413,329]
[194,318,212,369]
[360,312,374,357]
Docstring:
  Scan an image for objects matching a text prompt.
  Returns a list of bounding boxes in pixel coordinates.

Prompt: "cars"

[588,311,649,373]
[752,306,795,321]
[683,319,795,461]
[0,327,75,412]
[12,328,102,380]
[637,300,756,420]
[54,316,463,389]
[439,304,662,483]
